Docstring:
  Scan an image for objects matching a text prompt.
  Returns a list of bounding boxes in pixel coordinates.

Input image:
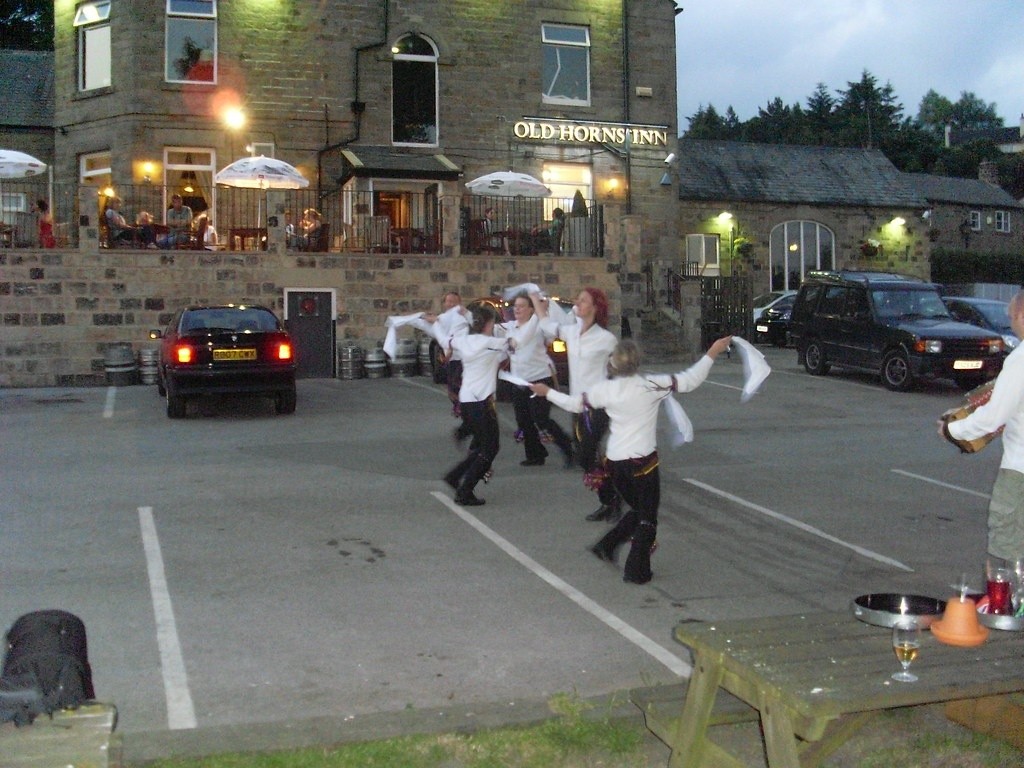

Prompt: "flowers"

[857,238,879,249]
[734,238,753,250]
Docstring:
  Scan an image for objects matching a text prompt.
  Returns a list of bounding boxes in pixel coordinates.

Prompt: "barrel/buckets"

[363,347,387,379]
[418,338,433,376]
[384,338,417,377]
[339,346,363,379]
[102,341,137,386]
[136,347,160,385]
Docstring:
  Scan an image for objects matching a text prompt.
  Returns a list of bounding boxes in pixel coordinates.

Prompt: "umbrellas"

[465,170,553,232]
[0,147,47,177]
[214,154,311,251]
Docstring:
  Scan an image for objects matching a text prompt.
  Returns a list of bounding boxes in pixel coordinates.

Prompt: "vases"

[861,246,877,257]
[738,243,751,254]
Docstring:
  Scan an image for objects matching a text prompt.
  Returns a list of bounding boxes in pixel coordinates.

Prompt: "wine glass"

[891,621,921,683]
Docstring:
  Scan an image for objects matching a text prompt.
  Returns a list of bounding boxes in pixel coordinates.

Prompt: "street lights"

[223,107,244,251]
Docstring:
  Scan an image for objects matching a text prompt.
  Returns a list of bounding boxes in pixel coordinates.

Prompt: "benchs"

[630,680,760,730]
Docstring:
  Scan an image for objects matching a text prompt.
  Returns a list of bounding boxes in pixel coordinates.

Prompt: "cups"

[985,557,1014,617]
[1005,557,1024,616]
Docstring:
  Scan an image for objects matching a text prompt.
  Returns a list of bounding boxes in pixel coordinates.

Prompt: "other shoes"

[520,457,545,466]
[590,545,614,562]
[624,571,653,584]
[442,475,459,490]
[456,498,486,506]
[562,449,579,469]
[585,504,621,523]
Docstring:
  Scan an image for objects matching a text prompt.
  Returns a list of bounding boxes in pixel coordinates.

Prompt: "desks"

[493,230,531,254]
[227,226,269,251]
[669,610,1024,768]
[129,224,170,248]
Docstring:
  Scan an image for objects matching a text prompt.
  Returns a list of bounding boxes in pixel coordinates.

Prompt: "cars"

[149,303,297,419]
[428,297,579,393]
[940,294,1022,358]
[751,288,796,346]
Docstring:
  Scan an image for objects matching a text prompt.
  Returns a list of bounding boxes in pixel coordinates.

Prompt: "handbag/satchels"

[0,610,96,728]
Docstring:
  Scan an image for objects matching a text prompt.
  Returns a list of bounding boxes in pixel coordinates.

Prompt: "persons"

[936,291,1024,561]
[286,208,326,246]
[135,211,153,224]
[390,291,524,505]
[529,290,625,524]
[27,199,57,249]
[477,208,511,256]
[183,39,214,82]
[457,293,577,470]
[105,197,160,250]
[157,193,193,249]
[529,207,566,256]
[191,213,220,246]
[529,335,733,584]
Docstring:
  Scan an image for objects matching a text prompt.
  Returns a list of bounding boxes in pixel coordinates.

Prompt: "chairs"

[187,318,205,329]
[0,218,559,250]
[239,319,257,332]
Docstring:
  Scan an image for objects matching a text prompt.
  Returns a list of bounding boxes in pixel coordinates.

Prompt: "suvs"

[787,271,1003,392]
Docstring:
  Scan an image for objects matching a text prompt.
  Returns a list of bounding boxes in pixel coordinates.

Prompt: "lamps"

[180,152,196,192]
